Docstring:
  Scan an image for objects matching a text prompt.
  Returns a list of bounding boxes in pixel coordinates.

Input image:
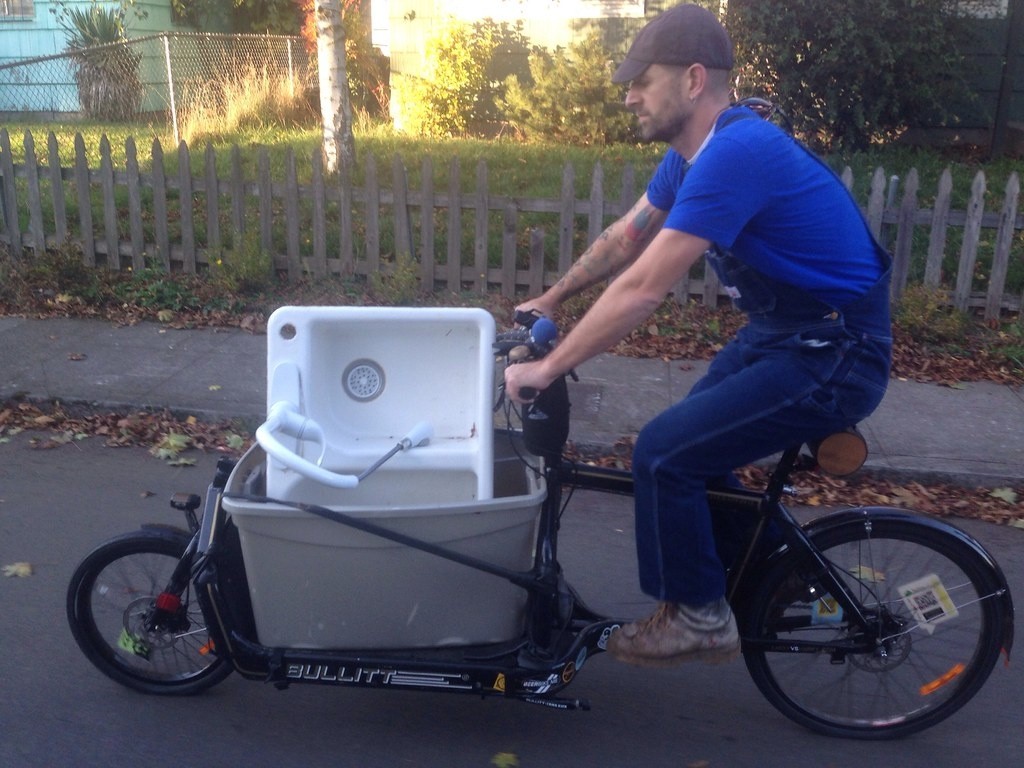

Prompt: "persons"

[504,4,892,669]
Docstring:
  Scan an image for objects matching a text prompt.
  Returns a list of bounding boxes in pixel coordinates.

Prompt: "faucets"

[255,397,435,491]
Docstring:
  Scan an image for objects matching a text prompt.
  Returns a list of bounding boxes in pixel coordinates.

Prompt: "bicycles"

[66,308,1014,745]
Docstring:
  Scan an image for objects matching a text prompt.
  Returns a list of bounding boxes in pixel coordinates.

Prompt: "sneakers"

[761,580,829,649]
[607,600,743,666]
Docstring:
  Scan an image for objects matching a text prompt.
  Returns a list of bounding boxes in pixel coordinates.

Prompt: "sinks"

[253,304,496,510]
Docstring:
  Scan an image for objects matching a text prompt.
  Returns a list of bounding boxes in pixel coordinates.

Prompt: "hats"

[610,5,733,84]
